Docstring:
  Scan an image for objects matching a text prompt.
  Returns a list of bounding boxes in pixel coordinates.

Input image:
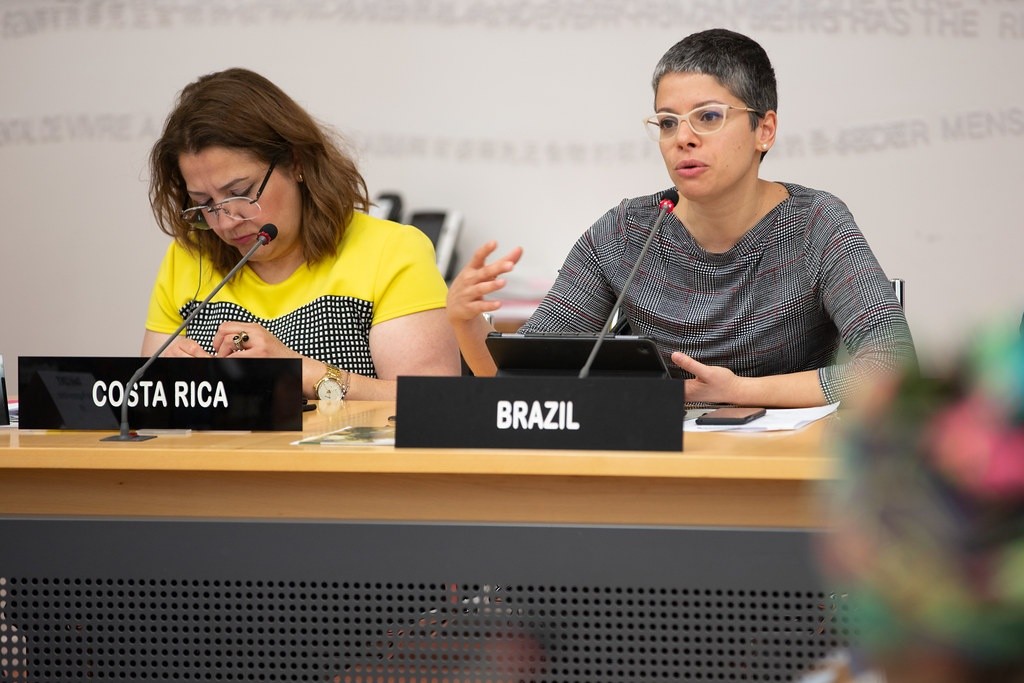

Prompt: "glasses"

[643,104,760,142]
[180,151,287,230]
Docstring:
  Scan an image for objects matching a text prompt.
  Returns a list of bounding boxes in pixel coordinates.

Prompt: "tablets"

[484,331,673,378]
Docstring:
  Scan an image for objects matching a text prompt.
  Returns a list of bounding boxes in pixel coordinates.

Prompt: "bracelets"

[346,371,351,392]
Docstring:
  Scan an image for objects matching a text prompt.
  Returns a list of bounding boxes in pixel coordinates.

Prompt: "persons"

[142,68,462,399]
[447,28,919,409]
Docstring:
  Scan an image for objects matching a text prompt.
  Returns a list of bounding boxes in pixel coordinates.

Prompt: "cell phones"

[695,407,766,425]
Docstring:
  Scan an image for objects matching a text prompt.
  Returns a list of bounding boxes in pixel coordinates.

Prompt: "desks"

[0,398,862,683]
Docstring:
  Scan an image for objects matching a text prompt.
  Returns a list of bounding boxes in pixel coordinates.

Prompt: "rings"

[233,331,249,350]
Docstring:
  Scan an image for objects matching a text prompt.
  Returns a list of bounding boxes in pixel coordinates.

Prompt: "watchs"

[313,363,346,400]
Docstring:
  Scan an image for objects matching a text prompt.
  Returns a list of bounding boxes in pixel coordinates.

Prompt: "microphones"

[98,223,279,441]
[577,190,680,378]
